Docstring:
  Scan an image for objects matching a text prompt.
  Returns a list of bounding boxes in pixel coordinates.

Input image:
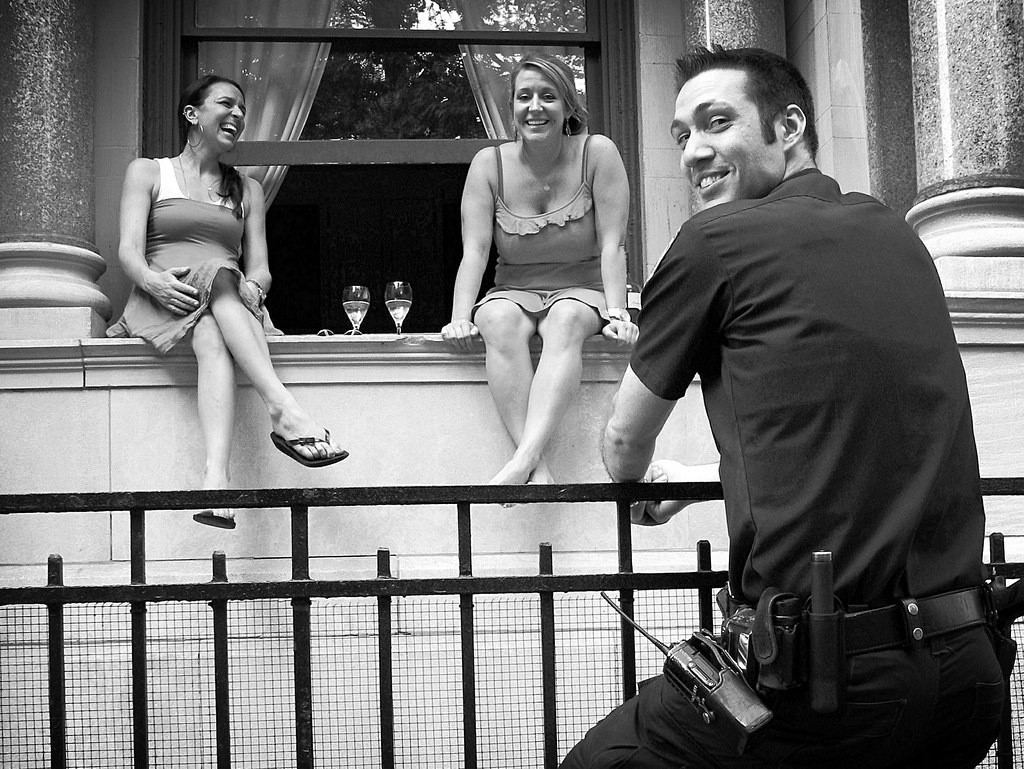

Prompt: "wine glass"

[342,285,370,331]
[384,281,413,335]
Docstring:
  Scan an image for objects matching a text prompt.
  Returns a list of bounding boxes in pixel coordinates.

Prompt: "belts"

[725,584,986,661]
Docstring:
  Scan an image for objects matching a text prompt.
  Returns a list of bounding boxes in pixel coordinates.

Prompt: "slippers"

[193,510,236,529]
[270,426,349,468]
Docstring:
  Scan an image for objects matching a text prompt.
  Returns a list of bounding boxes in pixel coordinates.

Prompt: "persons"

[107,71,352,532]
[556,46,1012,768]
[438,53,641,490]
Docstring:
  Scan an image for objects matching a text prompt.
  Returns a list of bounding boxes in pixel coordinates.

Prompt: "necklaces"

[519,137,564,193]
[178,153,222,198]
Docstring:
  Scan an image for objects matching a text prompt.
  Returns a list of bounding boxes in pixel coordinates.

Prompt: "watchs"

[607,308,631,322]
[247,278,267,308]
